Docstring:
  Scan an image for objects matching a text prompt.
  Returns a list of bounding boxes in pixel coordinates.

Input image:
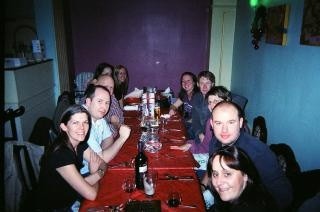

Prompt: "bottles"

[135,142,148,189]
[168,192,180,207]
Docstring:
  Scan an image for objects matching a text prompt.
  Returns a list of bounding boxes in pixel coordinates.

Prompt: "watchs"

[98,169,105,176]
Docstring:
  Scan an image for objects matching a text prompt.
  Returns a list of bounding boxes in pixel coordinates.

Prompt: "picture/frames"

[299,0,320,46]
[263,3,290,46]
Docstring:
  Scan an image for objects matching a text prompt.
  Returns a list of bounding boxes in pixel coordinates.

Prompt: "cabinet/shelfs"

[3,58,56,142]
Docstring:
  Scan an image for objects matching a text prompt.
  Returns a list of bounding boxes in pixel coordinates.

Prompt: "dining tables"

[106,104,194,169]
[78,169,207,212]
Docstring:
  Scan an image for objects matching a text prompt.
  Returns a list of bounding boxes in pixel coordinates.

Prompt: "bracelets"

[200,183,207,190]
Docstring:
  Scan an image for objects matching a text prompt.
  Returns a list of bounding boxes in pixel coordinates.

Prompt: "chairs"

[28,116,55,147]
[54,97,72,126]
[251,116,268,144]
[269,143,301,186]
[4,140,45,212]
[230,94,248,111]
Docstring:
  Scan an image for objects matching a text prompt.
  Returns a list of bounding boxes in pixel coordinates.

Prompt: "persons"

[79,85,131,178]
[206,146,276,212]
[112,64,129,95]
[200,101,293,212]
[170,86,232,154]
[169,71,200,128]
[32,104,108,212]
[78,63,125,108]
[79,74,124,138]
[187,71,249,144]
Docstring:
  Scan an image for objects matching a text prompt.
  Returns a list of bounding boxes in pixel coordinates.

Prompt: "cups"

[159,129,168,143]
[144,171,158,196]
[123,177,135,193]
[154,107,161,120]
